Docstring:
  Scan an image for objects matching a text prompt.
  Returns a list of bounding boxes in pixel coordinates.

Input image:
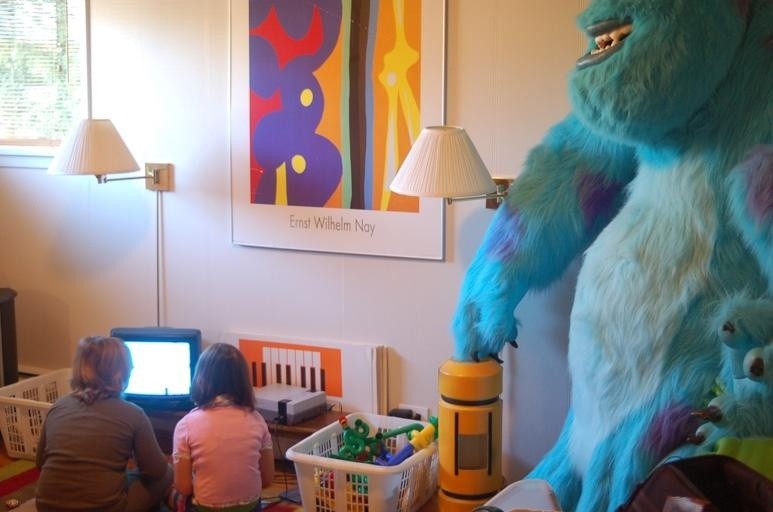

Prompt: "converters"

[389,409,412,419]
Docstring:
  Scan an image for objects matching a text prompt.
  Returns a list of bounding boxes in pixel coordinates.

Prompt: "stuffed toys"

[449,0,773,512]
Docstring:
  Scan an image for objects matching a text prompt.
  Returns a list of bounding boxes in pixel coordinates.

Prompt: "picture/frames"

[226,4,449,264]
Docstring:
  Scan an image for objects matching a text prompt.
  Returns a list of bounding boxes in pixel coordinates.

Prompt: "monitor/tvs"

[111,327,200,412]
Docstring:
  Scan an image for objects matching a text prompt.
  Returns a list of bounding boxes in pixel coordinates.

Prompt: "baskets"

[0,367,73,460]
[286,411,438,512]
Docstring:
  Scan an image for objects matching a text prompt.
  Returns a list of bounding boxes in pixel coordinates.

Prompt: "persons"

[164,343,275,512]
[36,336,174,512]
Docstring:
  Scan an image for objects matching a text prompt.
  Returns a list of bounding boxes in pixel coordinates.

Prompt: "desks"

[144,409,349,464]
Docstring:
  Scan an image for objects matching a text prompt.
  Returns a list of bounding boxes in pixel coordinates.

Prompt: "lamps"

[56,118,174,193]
[388,124,515,209]
